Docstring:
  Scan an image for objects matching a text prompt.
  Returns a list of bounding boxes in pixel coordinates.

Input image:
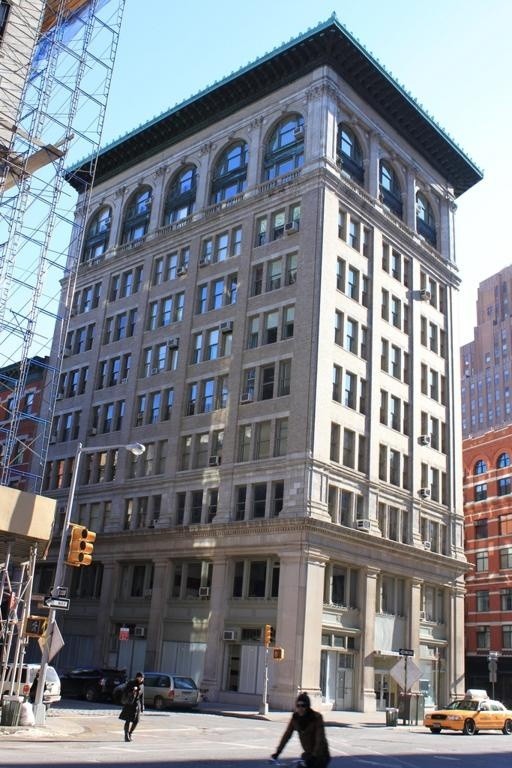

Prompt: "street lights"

[27,441,152,727]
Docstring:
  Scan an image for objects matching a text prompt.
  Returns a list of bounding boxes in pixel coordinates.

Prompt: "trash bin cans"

[385,707,399,727]
[2,695,24,726]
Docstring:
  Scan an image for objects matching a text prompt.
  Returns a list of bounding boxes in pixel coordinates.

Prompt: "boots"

[125,731,132,741]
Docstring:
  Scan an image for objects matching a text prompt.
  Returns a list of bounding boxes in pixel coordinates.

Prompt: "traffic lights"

[25,616,48,636]
[274,648,285,660]
[265,625,273,648]
[79,530,94,566]
[71,525,89,563]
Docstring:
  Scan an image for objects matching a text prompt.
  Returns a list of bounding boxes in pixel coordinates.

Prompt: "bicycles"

[267,756,306,768]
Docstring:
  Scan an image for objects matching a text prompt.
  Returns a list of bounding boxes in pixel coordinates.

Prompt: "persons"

[119,672,145,742]
[29,669,48,714]
[270,691,331,768]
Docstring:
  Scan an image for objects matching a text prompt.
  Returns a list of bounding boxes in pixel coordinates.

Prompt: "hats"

[296,694,311,706]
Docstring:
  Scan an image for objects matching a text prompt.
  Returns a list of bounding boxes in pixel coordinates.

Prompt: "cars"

[423,689,512,735]
[112,671,201,710]
[54,665,127,701]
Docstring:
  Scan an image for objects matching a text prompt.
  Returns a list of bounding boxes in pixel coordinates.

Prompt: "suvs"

[0,663,62,713]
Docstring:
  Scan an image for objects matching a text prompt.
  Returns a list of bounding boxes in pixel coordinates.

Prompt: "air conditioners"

[86,428,96,436]
[104,218,111,226]
[357,519,371,531]
[199,587,209,597]
[421,289,431,300]
[221,320,233,334]
[416,202,422,213]
[422,541,431,550]
[239,393,252,403]
[145,589,153,599]
[421,488,430,497]
[422,435,430,444]
[208,455,220,466]
[284,221,299,234]
[150,518,157,526]
[59,348,70,358]
[56,393,63,400]
[151,368,158,375]
[167,338,177,349]
[49,436,56,444]
[121,378,128,384]
[294,126,304,139]
[135,626,145,637]
[222,630,237,641]
[176,266,186,275]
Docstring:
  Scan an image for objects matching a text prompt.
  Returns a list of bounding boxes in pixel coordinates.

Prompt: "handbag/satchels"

[121,692,136,705]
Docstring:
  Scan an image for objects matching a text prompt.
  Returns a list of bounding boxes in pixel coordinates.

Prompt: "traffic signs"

[399,648,414,657]
[49,586,68,597]
[43,596,72,610]
